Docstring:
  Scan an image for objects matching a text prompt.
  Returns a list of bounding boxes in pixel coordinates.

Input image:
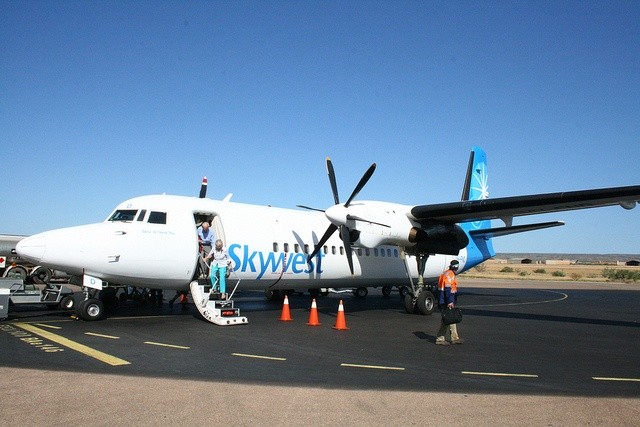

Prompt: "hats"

[449,260,459,268]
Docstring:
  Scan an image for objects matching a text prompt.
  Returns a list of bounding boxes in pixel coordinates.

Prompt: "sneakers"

[436,341,450,346]
[451,338,464,344]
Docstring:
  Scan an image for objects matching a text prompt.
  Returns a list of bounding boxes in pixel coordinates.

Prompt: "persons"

[203,240,232,300]
[435,260,465,345]
[197,222,214,279]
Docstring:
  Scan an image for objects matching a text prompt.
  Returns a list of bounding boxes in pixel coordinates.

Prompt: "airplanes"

[16,147,640,326]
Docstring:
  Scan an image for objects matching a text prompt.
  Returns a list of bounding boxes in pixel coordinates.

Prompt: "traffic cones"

[332,299,350,330]
[278,295,294,321]
[305,298,321,325]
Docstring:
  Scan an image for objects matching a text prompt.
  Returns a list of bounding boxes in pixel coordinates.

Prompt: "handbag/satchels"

[441,308,462,326]
